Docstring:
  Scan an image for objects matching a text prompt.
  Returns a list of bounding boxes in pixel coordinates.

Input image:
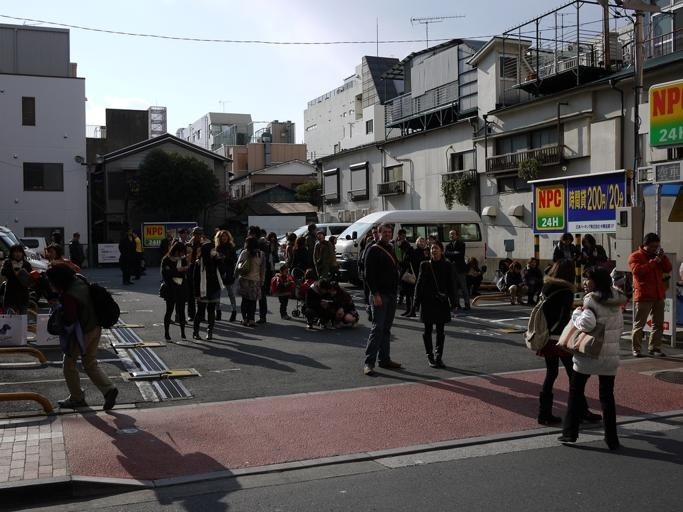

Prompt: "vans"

[270,208,488,288]
[0,225,55,304]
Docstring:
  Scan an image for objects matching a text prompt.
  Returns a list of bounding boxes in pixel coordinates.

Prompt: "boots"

[164,322,171,340]
[214,309,222,320]
[229,310,237,322]
[537,391,562,425]
[577,395,603,425]
[180,321,186,339]
[427,352,438,368]
[434,349,446,368]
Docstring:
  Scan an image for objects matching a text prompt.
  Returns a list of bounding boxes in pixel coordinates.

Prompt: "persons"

[47,260,118,411]
[537,262,602,426]
[69,233,85,267]
[47,232,65,260]
[119,230,135,285]
[47,243,81,273]
[557,264,627,451]
[132,231,144,280]
[0,245,32,315]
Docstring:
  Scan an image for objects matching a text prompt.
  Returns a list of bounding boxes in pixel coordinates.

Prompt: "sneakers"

[192,330,202,340]
[168,314,193,324]
[363,362,374,376]
[280,311,291,320]
[556,435,578,442]
[632,350,641,357]
[306,320,353,330]
[240,317,267,328]
[102,386,119,411]
[205,329,213,340]
[57,390,87,407]
[649,348,667,358]
[377,359,401,370]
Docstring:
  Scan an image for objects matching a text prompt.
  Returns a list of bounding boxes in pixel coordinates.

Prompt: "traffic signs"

[637,161,683,185]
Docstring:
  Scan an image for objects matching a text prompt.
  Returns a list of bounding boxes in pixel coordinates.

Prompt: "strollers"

[291,267,309,318]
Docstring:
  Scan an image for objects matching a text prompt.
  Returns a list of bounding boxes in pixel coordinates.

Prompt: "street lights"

[73,153,107,266]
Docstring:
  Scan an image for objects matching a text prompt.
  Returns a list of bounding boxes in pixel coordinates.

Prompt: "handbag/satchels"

[554,305,607,361]
[0,309,27,346]
[35,309,61,347]
[400,260,416,285]
[495,276,508,293]
[237,249,251,277]
[433,291,452,308]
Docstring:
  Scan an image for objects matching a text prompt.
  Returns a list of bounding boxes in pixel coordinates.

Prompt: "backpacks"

[74,272,122,330]
[523,285,571,352]
